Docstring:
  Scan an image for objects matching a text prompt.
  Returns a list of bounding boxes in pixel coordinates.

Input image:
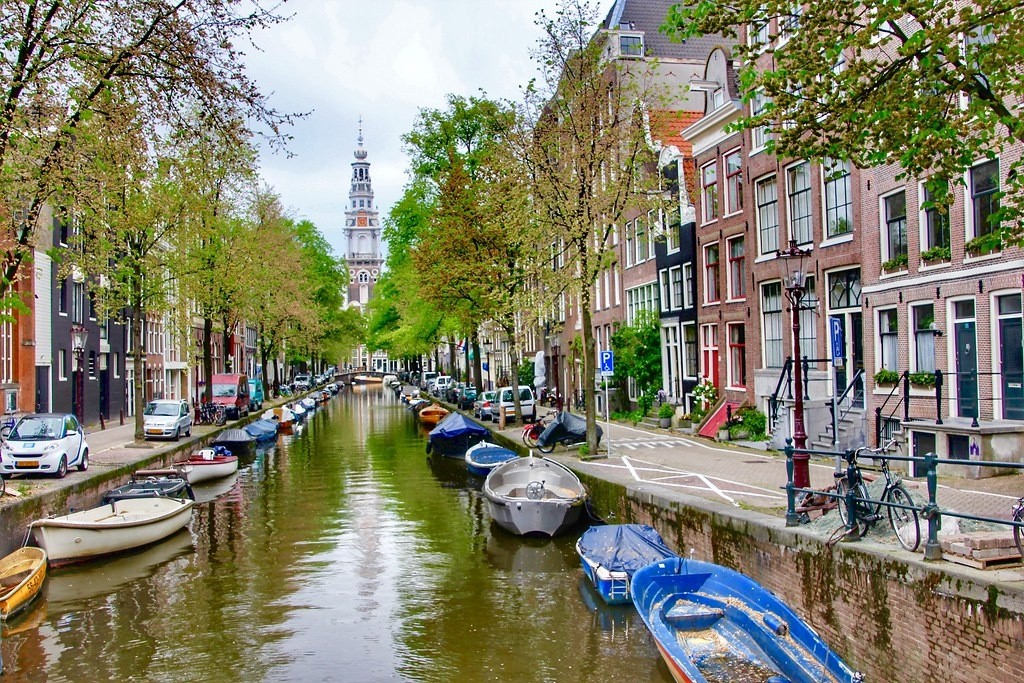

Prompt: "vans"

[201,372,250,420]
[490,385,536,424]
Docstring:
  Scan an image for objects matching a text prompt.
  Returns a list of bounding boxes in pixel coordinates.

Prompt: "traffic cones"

[193,403,201,426]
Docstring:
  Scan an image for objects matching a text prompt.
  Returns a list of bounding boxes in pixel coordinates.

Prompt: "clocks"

[358,217,367,226]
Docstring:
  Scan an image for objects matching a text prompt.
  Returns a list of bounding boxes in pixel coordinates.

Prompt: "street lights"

[546,328,563,412]
[775,238,812,515]
[70,322,89,426]
[483,337,492,391]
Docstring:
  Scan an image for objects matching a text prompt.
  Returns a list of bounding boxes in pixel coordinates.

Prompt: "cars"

[473,391,497,421]
[248,379,263,412]
[142,398,193,442]
[383,369,477,412]
[0,413,90,478]
[260,365,346,429]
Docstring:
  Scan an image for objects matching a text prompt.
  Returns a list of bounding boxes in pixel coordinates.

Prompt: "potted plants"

[872,369,898,387]
[921,243,942,265]
[900,254,908,270]
[631,411,641,427]
[600,382,613,389]
[942,248,951,263]
[720,424,729,443]
[911,371,935,389]
[657,401,674,429]
[966,233,1001,256]
[883,257,900,273]
[692,416,701,432]
[730,425,750,440]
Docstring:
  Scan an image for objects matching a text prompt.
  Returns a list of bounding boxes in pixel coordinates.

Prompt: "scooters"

[201,401,228,427]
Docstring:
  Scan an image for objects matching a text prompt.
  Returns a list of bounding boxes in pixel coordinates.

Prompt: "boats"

[419,401,450,428]
[428,411,491,460]
[630,556,867,683]
[134,445,240,487]
[575,524,686,606]
[465,439,522,478]
[24,497,197,570]
[100,463,192,515]
[242,417,280,442]
[208,427,258,455]
[0,546,50,625]
[482,455,588,539]
[189,471,241,505]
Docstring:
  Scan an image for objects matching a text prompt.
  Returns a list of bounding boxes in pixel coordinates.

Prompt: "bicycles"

[1011,496,1024,560]
[834,439,920,552]
[0,404,41,442]
[521,410,603,453]
[0,474,6,499]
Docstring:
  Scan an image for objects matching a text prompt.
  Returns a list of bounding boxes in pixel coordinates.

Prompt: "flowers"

[694,383,720,403]
